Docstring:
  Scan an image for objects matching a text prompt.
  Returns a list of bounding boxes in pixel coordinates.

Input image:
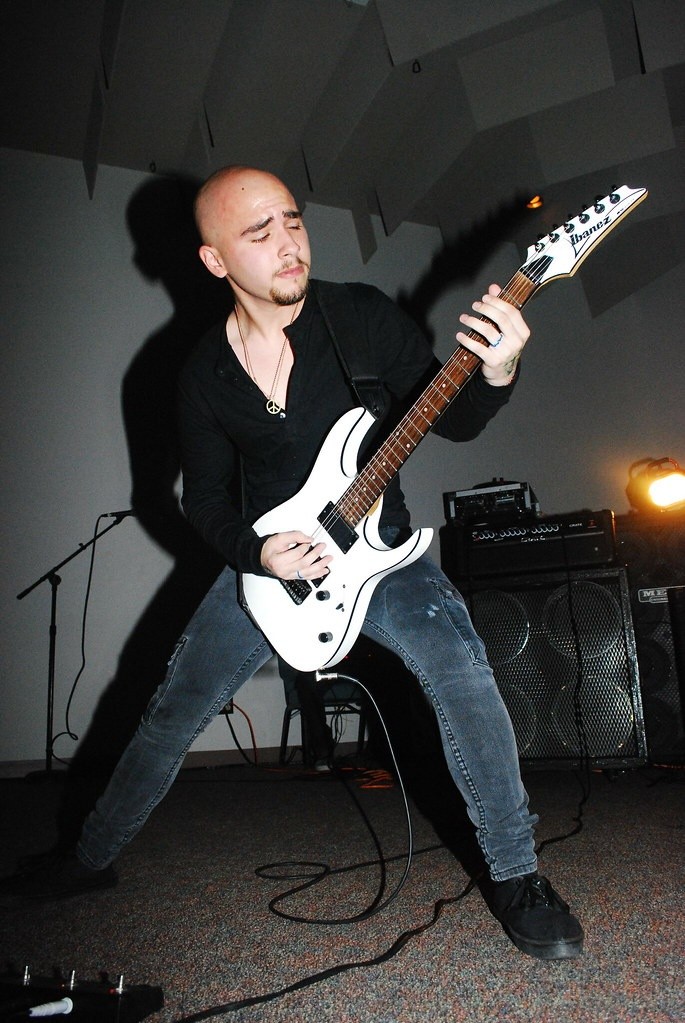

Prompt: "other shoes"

[0,846,119,904]
[493,874,584,959]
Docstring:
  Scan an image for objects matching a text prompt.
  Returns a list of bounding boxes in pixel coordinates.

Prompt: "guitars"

[238,185,649,674]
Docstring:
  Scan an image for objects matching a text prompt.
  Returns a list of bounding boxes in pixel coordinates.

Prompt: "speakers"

[451,573,646,781]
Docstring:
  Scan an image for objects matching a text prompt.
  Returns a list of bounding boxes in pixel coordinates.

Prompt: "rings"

[493,334,502,347]
[298,571,302,578]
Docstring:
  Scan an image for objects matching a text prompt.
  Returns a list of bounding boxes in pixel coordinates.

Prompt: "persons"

[0,165,583,962]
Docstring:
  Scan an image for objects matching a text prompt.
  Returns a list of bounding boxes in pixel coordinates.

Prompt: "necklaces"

[234,303,298,414]
